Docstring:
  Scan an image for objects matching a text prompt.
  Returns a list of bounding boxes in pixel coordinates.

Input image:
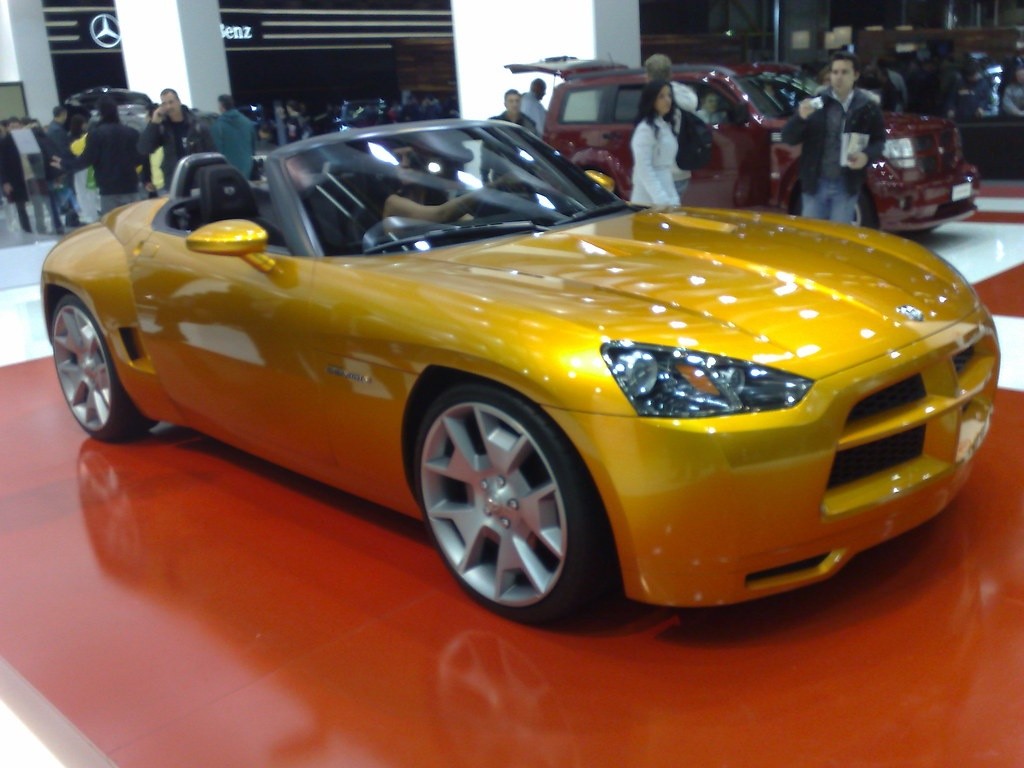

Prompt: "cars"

[501,54,981,234]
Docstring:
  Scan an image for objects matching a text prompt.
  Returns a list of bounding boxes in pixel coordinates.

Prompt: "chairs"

[170,151,282,253]
[310,162,384,249]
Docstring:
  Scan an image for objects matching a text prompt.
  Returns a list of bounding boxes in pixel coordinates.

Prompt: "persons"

[0,79,550,248]
[630,50,1024,225]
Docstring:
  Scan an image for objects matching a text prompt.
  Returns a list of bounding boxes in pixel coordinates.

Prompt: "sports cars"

[40,117,1004,633]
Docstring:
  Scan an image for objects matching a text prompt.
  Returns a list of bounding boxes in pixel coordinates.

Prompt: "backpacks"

[671,104,713,170]
